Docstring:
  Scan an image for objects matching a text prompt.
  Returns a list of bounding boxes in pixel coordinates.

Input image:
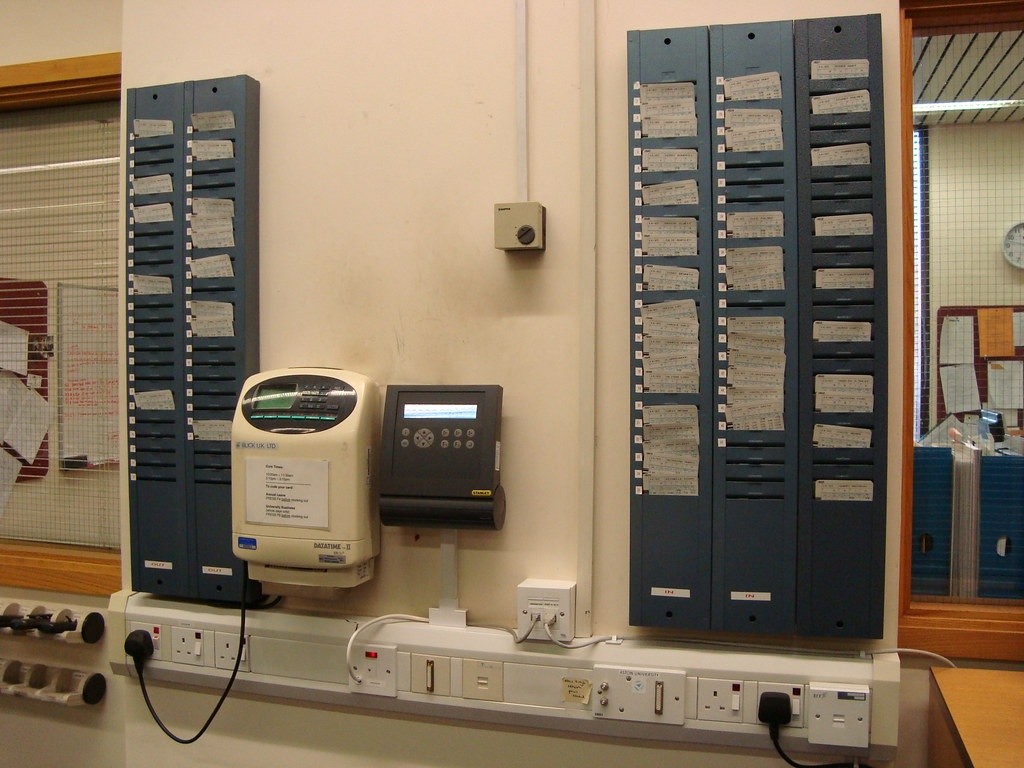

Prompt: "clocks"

[1001,222,1024,271]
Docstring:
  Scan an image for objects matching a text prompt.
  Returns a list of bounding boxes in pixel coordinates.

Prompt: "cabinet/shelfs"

[923,664,1024,767]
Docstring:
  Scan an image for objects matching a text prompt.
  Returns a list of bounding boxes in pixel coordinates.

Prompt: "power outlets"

[214,631,251,673]
[756,681,807,728]
[170,625,206,668]
[697,677,744,722]
[130,621,163,661]
